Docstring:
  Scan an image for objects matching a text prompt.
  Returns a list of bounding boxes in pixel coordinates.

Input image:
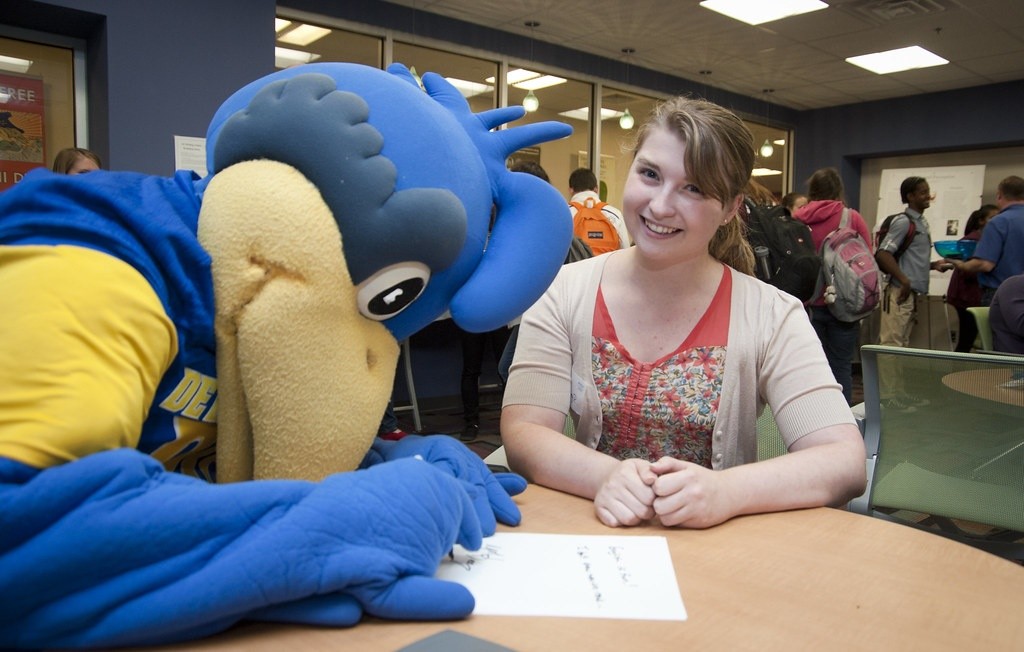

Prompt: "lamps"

[760,88,774,157]
[523,21,539,112]
[619,49,635,129]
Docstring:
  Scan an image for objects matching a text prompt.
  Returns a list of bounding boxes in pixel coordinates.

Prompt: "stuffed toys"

[0,61,573,652]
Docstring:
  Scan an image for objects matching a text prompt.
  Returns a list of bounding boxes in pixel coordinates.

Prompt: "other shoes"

[381,431,407,440]
[460,422,479,441]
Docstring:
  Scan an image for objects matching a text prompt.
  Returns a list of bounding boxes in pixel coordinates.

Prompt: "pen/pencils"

[414,453,455,563]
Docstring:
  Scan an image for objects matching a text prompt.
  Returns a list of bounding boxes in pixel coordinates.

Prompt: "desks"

[122,483,1023,650]
[938,370,1024,419]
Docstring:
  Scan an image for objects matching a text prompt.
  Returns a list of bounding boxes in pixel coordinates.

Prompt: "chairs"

[487,344,1022,568]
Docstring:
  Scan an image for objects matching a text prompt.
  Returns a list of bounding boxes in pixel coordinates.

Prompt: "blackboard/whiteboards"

[578,150,625,207]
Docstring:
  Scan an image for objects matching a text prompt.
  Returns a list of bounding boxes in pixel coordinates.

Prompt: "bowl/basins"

[933,240,978,263]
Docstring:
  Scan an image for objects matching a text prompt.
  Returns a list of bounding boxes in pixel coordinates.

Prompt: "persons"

[499,96,866,529]
[498,161,551,387]
[568,167,631,256]
[874,176,954,413]
[377,393,408,441]
[782,192,808,216]
[452,319,512,443]
[736,178,780,284]
[989,274,1024,354]
[944,176,1024,307]
[52,148,102,174]
[791,167,873,408]
[946,204,1000,353]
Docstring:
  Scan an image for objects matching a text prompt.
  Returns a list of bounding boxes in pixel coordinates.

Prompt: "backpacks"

[804,207,881,322]
[871,213,915,266]
[568,197,619,257]
[743,194,820,302]
